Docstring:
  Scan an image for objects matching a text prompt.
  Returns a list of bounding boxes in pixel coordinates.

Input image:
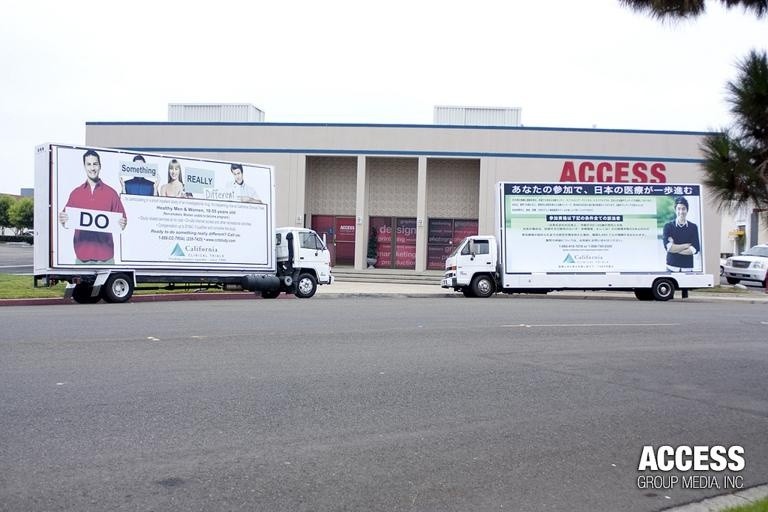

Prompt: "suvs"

[723,241,767,291]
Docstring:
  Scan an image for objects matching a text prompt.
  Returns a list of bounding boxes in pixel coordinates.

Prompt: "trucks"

[439,180,714,304]
[31,143,340,307]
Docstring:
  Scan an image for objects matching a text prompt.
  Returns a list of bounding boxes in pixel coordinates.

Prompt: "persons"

[663,196,703,272]
[58,149,128,265]
[115,156,162,198]
[159,159,190,198]
[228,164,262,203]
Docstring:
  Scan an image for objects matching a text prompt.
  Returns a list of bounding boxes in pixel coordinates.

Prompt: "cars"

[719,259,726,277]
